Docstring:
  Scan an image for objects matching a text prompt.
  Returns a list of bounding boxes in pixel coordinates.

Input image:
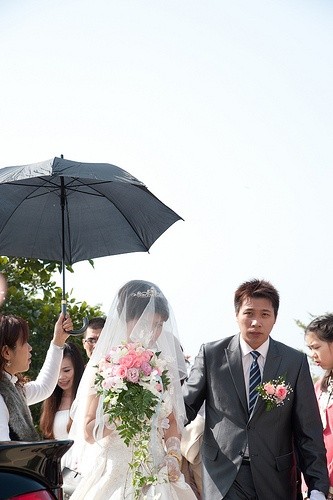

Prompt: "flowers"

[255,371,294,417]
[90,340,171,500]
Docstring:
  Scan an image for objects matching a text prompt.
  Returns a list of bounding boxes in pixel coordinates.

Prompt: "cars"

[0,439,74,500]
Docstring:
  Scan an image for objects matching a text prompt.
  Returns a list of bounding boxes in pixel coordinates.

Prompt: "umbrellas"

[0,154,185,336]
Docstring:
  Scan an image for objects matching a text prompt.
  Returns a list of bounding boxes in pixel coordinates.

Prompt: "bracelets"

[168,450,182,462]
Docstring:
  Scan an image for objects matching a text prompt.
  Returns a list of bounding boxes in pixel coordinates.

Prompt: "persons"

[83,317,106,359]
[40,342,85,500]
[67,280,197,500]
[0,311,74,440]
[301,313,333,500]
[181,280,331,500]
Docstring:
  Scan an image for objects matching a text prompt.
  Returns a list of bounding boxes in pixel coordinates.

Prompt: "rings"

[173,475,178,479]
